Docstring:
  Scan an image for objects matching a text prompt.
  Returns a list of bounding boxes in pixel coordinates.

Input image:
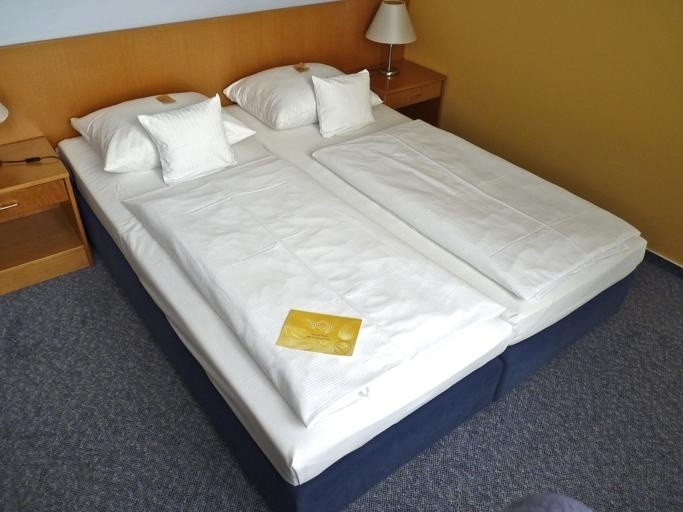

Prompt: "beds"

[56,108,513,511]
[224,105,645,401]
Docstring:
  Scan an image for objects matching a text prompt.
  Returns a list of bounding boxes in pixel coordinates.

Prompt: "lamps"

[364,1,419,79]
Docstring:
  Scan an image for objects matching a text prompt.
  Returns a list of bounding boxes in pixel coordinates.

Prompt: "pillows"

[69,92,256,174]
[311,69,377,138]
[222,62,384,127]
[138,93,236,187]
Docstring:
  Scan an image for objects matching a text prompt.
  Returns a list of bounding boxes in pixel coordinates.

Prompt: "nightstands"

[0,136,95,297]
[345,58,447,128]
